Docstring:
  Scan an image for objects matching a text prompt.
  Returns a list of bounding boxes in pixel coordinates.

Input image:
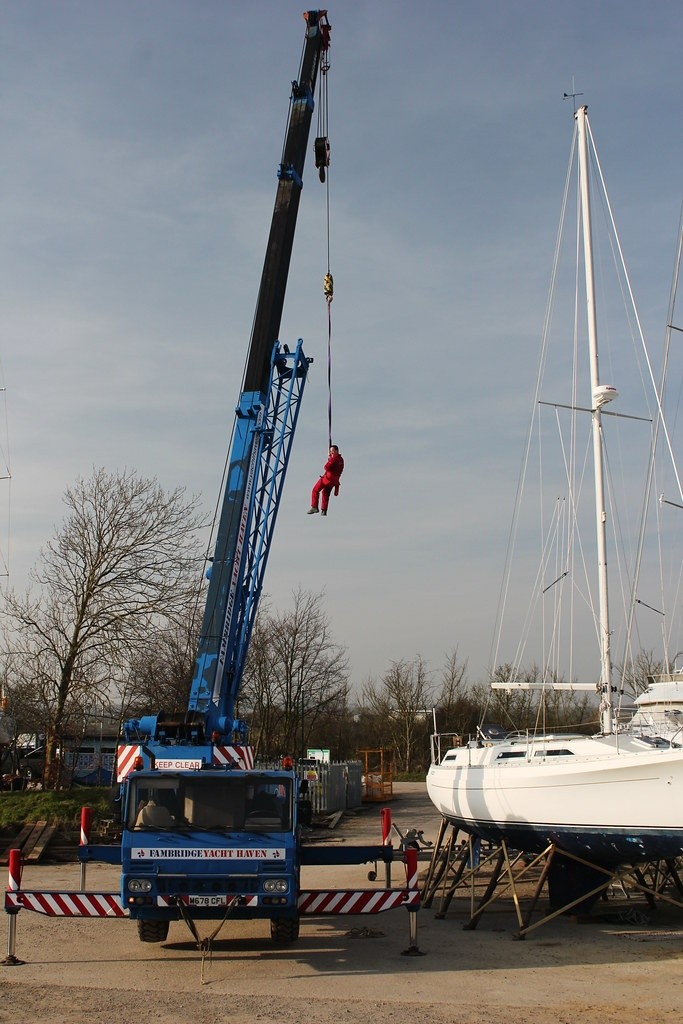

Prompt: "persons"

[307,445,344,517]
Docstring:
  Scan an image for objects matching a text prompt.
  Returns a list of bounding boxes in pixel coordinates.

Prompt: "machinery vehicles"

[111,9,333,947]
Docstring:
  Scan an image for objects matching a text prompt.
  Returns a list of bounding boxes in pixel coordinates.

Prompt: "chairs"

[246,792,284,829]
[135,805,174,826]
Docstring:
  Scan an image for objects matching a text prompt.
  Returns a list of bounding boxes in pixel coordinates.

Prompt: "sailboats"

[425,77,683,914]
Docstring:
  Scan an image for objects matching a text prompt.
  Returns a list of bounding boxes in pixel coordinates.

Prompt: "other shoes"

[307,507,319,514]
[321,510,326,516]
